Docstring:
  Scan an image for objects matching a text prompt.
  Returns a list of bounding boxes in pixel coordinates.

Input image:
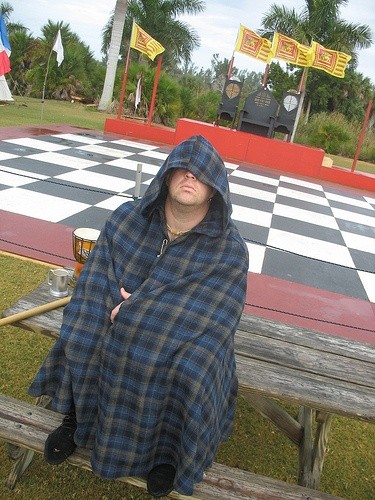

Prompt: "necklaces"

[166,225,189,235]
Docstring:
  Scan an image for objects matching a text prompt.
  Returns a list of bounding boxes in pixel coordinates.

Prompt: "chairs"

[237,88,279,139]
[271,90,303,142]
[216,74,244,129]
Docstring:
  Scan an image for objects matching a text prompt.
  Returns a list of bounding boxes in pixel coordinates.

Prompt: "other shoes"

[43,412,77,466]
[146,465,176,498]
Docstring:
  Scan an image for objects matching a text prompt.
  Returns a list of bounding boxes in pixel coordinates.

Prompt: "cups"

[48,269,69,297]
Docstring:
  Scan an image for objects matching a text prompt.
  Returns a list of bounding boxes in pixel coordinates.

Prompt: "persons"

[29,135,249,497]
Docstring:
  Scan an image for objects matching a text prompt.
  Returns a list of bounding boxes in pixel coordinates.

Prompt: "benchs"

[0,393,347,500]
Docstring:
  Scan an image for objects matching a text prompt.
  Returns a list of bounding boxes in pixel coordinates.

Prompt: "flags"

[273,33,300,64]
[135,79,141,109]
[130,23,166,61]
[311,41,352,78]
[235,24,274,64]
[53,29,64,67]
[298,44,310,67]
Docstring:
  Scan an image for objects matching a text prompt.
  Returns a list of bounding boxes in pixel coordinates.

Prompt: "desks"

[0,270,375,427]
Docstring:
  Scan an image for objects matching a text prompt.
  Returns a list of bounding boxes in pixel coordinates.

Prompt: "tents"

[0,12,15,102]
